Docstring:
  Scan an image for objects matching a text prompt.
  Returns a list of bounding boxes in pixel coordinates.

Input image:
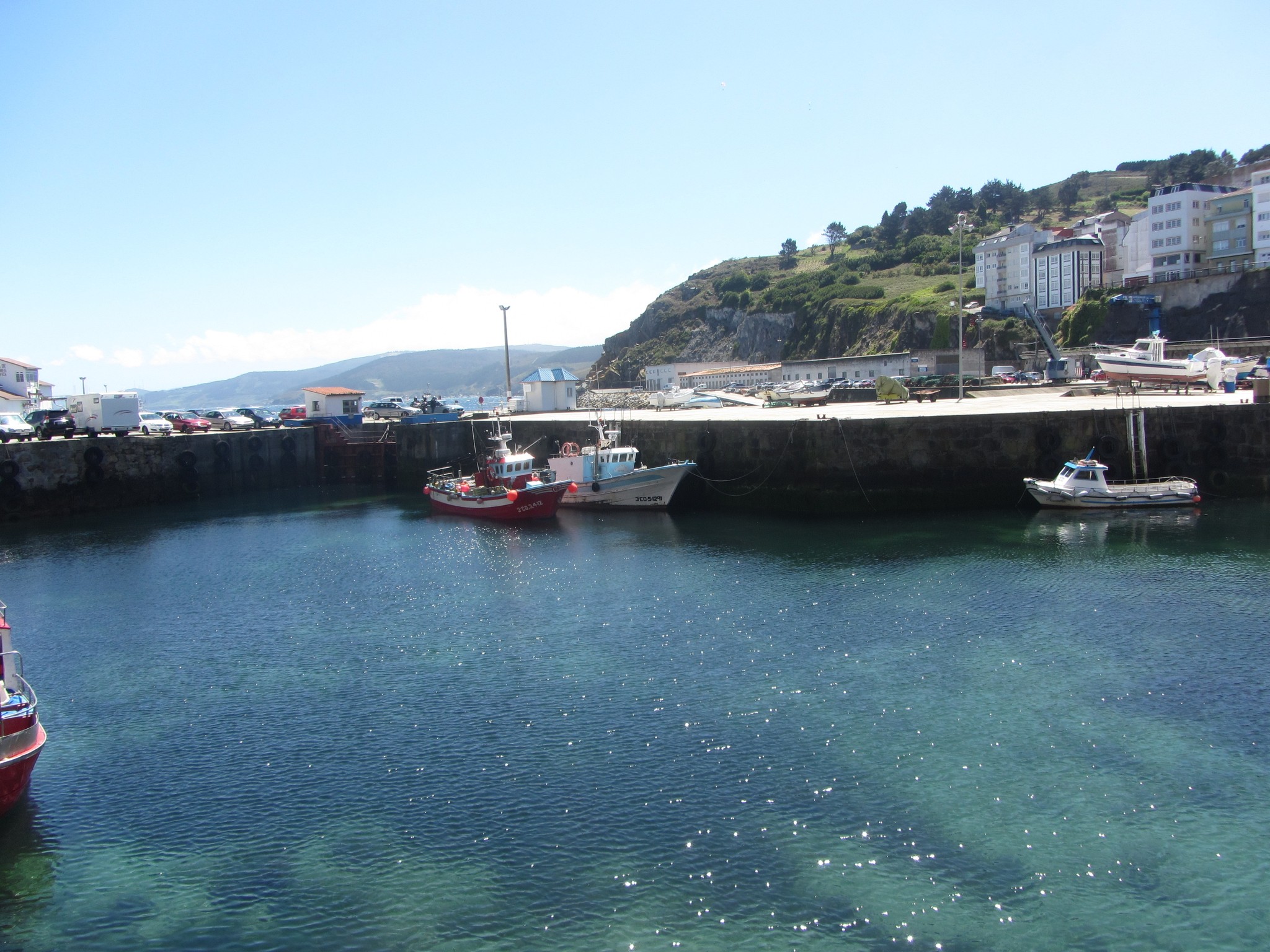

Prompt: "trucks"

[66,392,141,438]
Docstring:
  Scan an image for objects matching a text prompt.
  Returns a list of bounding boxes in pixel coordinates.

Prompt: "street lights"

[104,384,107,393]
[947,213,974,404]
[79,377,87,394]
[499,304,513,413]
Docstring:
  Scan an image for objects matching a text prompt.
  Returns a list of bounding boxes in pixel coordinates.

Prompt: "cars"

[236,407,283,430]
[279,406,306,425]
[122,412,174,436]
[186,409,211,417]
[719,375,911,406]
[991,365,1043,384]
[426,400,464,417]
[162,412,212,433]
[0,412,35,443]
[366,401,423,420]
[154,410,178,418]
[199,410,255,431]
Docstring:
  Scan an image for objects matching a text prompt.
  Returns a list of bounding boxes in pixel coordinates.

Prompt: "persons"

[412,396,438,413]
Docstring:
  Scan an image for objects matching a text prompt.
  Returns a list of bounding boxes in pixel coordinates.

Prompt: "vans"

[693,384,707,390]
[380,397,404,408]
[630,386,643,392]
[663,383,676,391]
[1090,369,1109,381]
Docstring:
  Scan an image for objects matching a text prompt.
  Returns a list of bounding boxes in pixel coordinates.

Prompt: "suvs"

[17,409,76,442]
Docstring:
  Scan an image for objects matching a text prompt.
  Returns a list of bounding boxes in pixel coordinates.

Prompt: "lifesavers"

[1096,435,1122,480]
[592,482,598,492]
[486,467,495,481]
[364,407,371,415]
[215,440,230,472]
[249,454,264,470]
[1162,437,1186,479]
[84,446,105,483]
[0,459,20,500]
[179,449,199,484]
[696,430,715,470]
[1037,428,1061,476]
[1203,420,1230,490]
[282,435,296,465]
[561,442,580,457]
[247,435,262,451]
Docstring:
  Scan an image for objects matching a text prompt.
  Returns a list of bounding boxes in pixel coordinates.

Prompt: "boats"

[424,412,578,521]
[0,600,48,816]
[546,362,698,512]
[1088,325,1270,384]
[647,388,723,408]
[1022,446,1201,507]
